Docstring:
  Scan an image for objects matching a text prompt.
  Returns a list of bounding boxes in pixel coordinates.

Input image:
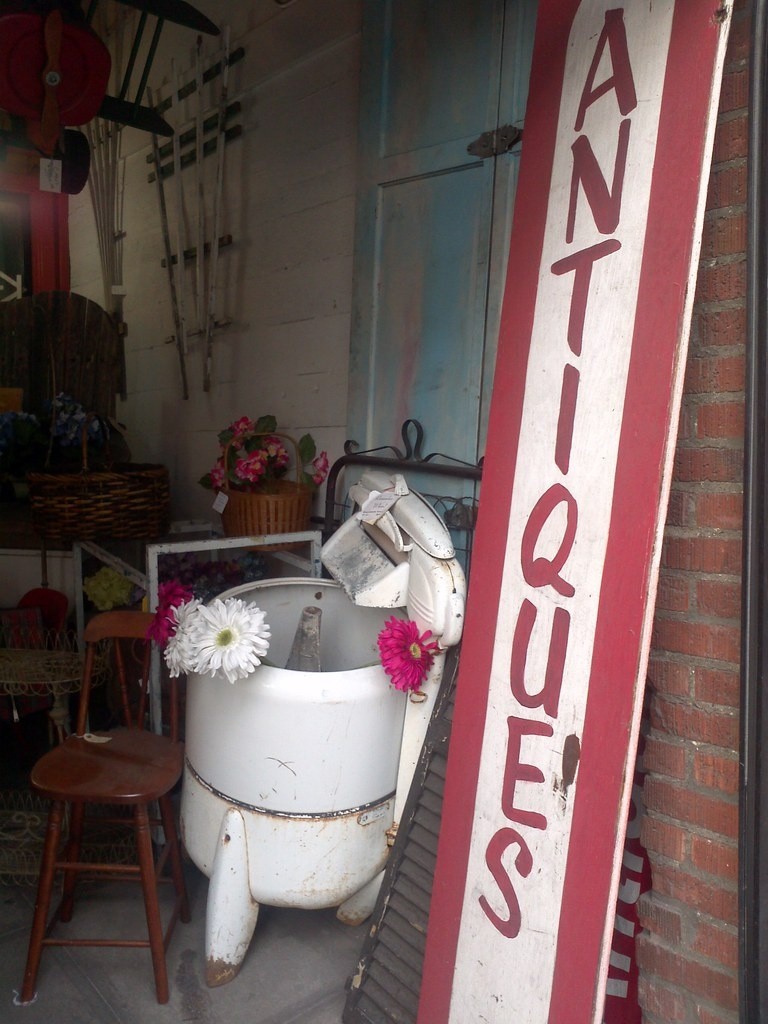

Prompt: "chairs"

[22,609,192,1004]
[18,587,69,649]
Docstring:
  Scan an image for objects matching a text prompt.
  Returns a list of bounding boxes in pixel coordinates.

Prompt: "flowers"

[1,391,329,684]
[353,616,440,694]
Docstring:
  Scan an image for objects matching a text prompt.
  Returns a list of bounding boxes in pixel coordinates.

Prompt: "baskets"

[25,411,171,542]
[220,433,313,551]
